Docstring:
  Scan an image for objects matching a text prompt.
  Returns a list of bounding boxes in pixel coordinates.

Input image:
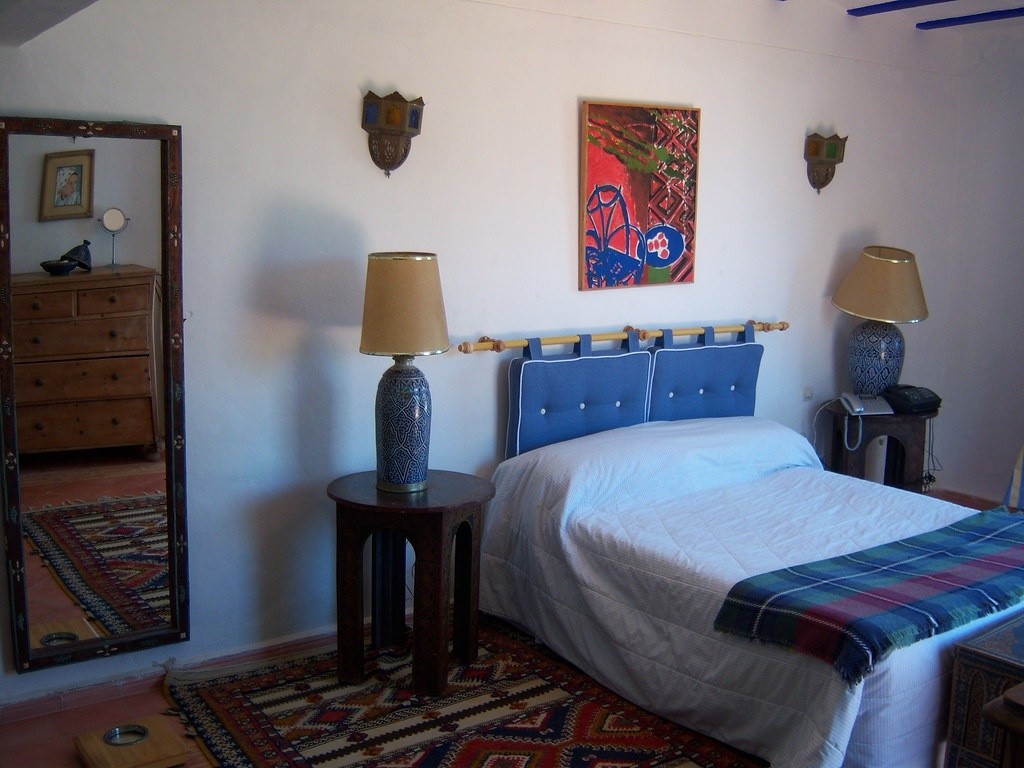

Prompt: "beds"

[460,320,1023,768]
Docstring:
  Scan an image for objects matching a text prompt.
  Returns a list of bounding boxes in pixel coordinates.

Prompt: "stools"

[940,618,1024,768]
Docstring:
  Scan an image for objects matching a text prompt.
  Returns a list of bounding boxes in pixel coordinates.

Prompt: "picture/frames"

[577,100,702,292]
[39,147,95,221]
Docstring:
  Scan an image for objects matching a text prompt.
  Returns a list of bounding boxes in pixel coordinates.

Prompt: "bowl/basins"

[40,259,79,276]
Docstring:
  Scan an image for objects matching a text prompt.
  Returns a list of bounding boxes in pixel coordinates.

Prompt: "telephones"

[841,393,894,416]
[890,384,942,413]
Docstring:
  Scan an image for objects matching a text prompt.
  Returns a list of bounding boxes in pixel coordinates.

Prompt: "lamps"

[361,89,425,178]
[358,251,453,492]
[830,246,930,396]
[804,131,848,195]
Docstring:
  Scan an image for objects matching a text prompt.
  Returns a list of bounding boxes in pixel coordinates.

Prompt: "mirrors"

[0,118,191,674]
[98,208,131,267]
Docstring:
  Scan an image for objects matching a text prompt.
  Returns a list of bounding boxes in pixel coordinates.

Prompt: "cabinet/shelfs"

[9,261,164,463]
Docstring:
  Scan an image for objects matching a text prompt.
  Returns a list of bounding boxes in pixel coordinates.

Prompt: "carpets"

[163,615,766,768]
[21,488,174,640]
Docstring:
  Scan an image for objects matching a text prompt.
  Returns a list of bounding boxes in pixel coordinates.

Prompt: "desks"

[820,397,939,493]
[332,467,495,695]
